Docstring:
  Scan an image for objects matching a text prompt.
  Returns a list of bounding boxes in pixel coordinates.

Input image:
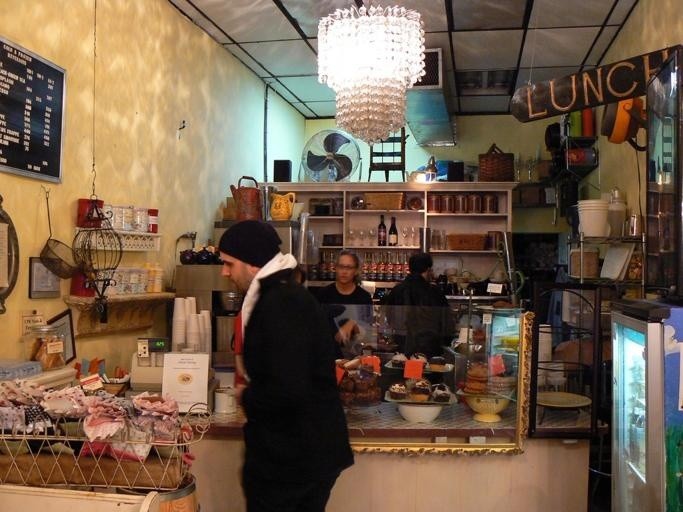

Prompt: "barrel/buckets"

[577,200,608,237]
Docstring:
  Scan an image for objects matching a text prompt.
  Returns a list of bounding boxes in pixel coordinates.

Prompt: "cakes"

[416,379,430,390]
[392,352,408,367]
[432,388,449,401]
[429,357,447,371]
[335,357,381,402]
[465,362,499,381]
[408,386,430,401]
[389,383,406,398]
[485,377,516,396]
[410,352,428,369]
[459,379,485,393]
[432,383,447,391]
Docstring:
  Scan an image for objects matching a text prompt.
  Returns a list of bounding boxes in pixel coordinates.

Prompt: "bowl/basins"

[397,404,443,424]
[464,396,510,423]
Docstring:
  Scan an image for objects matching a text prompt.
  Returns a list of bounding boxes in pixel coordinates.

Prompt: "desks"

[102,372,610,512]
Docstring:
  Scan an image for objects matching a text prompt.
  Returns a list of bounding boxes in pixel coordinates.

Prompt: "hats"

[217,218,283,268]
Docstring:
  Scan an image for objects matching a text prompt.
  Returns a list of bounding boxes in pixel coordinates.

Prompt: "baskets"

[477,143,515,181]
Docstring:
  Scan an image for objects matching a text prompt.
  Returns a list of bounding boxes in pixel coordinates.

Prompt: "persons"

[219,220,354,512]
[386,253,455,384]
[316,250,373,359]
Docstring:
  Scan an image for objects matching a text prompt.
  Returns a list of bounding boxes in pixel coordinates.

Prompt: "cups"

[368,226,377,247]
[400,225,409,247]
[346,228,355,246]
[357,227,366,247]
[215,389,237,415]
[412,225,419,247]
[171,297,211,354]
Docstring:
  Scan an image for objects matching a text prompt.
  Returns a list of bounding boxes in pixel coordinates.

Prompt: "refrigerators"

[610,298,683,512]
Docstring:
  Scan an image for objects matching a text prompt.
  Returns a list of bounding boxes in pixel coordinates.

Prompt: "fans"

[299,129,360,182]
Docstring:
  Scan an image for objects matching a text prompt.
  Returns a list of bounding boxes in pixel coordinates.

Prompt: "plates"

[464,387,515,393]
[384,360,454,372]
[536,392,591,408]
[384,390,457,404]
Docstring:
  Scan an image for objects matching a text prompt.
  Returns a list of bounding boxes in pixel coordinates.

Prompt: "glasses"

[335,264,355,269]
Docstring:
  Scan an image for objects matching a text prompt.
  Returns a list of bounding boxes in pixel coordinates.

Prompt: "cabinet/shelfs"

[253,182,519,363]
[566,231,647,457]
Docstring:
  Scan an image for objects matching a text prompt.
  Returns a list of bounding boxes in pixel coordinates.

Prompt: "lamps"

[316,2,425,144]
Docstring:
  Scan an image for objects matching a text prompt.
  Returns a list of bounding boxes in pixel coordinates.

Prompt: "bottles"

[432,229,441,251]
[395,249,403,281]
[389,217,398,245]
[403,251,410,279]
[608,186,628,237]
[628,407,639,462]
[319,248,329,280]
[387,250,394,283]
[370,252,377,280]
[329,251,336,279]
[635,410,645,473]
[144,262,155,292]
[379,214,387,246]
[378,250,385,282]
[154,263,163,292]
[361,250,368,280]
